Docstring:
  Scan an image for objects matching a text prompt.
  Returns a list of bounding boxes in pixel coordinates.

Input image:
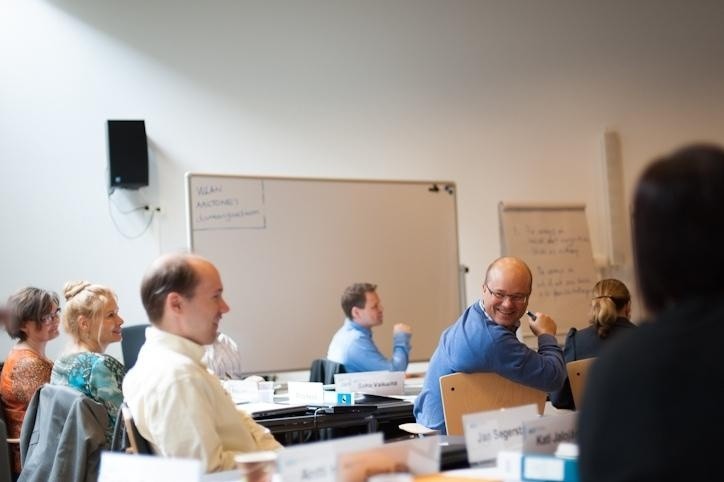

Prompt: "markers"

[526,310,537,319]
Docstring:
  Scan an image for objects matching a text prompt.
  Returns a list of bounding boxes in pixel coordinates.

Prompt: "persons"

[327,282,413,373]
[548,278,639,412]
[49,281,125,449]
[200,332,242,380]
[577,143,723,482]
[0,286,61,481]
[413,258,567,437]
[121,252,285,475]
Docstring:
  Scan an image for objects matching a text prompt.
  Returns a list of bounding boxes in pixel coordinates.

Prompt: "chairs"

[565,354,601,410]
[120,323,152,373]
[310,359,347,386]
[120,401,142,455]
[439,371,549,435]
[33,381,99,482]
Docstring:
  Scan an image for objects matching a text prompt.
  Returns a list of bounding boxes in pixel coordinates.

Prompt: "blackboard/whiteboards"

[185,171,462,375]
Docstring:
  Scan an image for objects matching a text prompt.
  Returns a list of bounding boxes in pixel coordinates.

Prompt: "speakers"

[108,120,148,187]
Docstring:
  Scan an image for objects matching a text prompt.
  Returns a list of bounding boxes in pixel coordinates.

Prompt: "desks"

[217,361,433,448]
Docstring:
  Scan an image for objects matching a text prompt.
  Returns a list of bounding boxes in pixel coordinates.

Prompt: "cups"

[235,450,277,482]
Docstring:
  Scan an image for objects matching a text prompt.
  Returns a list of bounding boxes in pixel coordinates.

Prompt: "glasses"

[19,309,61,324]
[482,284,530,304]
